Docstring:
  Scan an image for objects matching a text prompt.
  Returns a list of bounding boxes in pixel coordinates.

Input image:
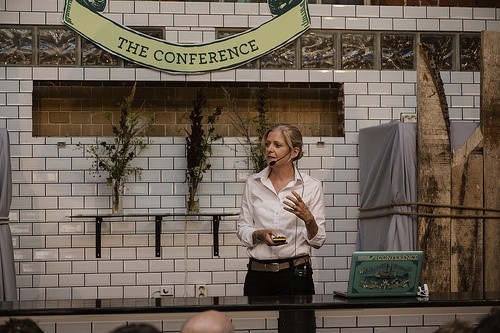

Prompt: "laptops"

[332,250,424,298]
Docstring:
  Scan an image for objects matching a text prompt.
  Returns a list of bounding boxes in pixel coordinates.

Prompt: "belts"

[251,256,309,273]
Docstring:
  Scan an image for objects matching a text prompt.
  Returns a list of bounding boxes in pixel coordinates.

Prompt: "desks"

[0,291,500,333]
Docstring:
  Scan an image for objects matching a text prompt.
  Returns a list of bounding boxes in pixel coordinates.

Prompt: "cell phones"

[272,236,286,242]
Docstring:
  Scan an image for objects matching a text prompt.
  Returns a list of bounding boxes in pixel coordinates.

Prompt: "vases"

[187,201,199,215]
[113,201,123,214]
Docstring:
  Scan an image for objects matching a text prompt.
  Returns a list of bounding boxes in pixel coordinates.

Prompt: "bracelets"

[305,217,314,226]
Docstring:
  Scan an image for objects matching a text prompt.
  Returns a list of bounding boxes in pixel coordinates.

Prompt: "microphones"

[269,148,292,166]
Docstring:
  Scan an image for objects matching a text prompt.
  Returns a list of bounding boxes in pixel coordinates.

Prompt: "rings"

[293,205,296,209]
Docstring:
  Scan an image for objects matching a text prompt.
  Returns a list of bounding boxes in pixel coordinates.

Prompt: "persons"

[181,310,235,333]
[109,322,162,333]
[0,317,43,333]
[236,123,326,296]
